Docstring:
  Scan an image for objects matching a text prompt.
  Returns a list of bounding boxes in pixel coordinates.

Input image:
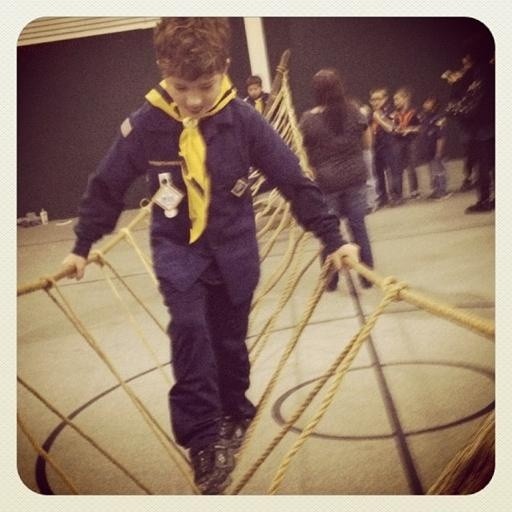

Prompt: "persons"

[62,19,363,496]
[297,67,377,290]
[241,75,270,112]
[448,38,496,217]
[347,86,480,214]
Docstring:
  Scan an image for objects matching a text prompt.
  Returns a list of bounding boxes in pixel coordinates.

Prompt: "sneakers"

[189,406,250,493]
[464,197,495,217]
[372,183,493,207]
[325,271,339,291]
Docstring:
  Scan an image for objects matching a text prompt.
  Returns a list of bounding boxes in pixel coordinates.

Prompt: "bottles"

[40,208,49,224]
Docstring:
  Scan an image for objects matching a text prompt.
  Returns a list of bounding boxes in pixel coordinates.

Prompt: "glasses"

[369,97,383,103]
